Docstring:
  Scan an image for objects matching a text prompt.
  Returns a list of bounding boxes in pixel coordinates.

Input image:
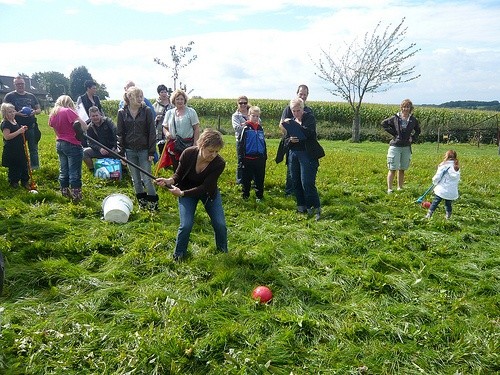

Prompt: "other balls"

[252,285,273,301]
[422,202,430,208]
[28,190,39,194]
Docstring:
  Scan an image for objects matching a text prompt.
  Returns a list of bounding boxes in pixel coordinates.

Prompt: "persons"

[162,89,200,174]
[277,84,314,197]
[48,94,89,204]
[81,104,127,174]
[120,79,159,164]
[152,84,174,158]
[153,127,229,260]
[424,148,460,220]
[4,76,40,168]
[114,86,158,210]
[232,96,261,183]
[236,106,267,201]
[284,98,324,221]
[76,79,106,145]
[381,98,421,193]
[0,103,38,191]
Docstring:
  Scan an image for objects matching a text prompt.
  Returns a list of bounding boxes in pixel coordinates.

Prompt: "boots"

[136,192,147,212]
[61,186,71,198]
[146,194,159,211]
[71,186,83,205]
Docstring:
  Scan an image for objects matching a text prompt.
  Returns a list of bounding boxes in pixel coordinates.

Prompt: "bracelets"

[179,191,182,198]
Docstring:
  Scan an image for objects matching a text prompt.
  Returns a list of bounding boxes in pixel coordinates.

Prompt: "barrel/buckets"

[102,193,131,221]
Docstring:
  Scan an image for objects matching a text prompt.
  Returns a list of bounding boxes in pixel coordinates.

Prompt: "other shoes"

[21,181,31,190]
[11,182,20,190]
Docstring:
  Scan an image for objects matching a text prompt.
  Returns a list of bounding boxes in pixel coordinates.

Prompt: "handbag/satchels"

[174,134,195,154]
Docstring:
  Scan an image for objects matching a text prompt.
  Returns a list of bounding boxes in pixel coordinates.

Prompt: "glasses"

[239,102,247,105]
[15,83,24,86]
